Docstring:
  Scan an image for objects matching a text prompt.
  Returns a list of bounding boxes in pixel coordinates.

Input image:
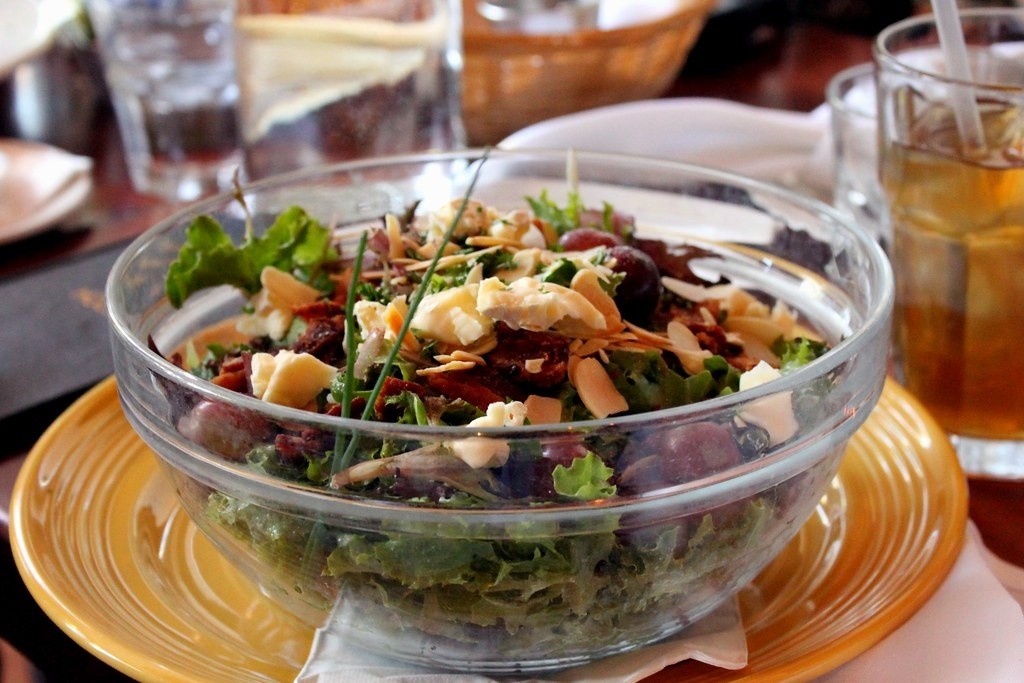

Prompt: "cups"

[88,0,244,199]
[869,4,1021,485]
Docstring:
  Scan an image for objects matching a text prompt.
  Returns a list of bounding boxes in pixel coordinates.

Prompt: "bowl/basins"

[105,141,899,668]
[825,49,889,253]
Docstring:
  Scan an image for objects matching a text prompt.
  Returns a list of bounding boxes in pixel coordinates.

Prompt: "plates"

[0,127,92,257]
[5,359,968,681]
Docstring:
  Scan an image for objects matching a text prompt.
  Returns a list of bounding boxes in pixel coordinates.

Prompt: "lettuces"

[120,164,857,660]
